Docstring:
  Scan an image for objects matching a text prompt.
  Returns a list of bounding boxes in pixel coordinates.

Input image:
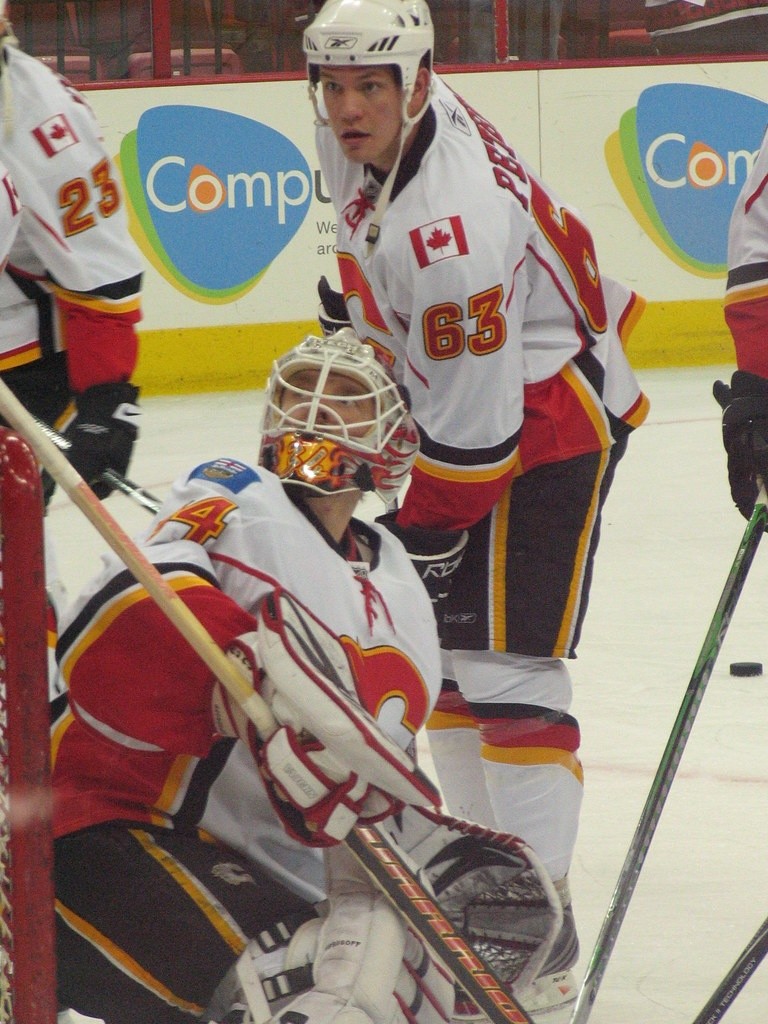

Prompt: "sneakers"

[453,877,578,1020]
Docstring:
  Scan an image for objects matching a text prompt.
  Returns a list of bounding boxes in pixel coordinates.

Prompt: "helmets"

[257,326,421,502]
[302,0,435,126]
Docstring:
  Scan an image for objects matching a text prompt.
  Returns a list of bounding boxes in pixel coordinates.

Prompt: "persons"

[304,0,650,1006]
[50,333,563,1024]
[0,0,147,728]
[713,128,768,532]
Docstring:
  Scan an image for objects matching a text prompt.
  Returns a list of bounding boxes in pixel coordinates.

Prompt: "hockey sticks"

[0,375,536,1024]
[559,486,768,1023]
[27,408,163,516]
[679,920,768,1024]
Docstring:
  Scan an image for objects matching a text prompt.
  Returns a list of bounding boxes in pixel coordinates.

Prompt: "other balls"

[731,661,763,678]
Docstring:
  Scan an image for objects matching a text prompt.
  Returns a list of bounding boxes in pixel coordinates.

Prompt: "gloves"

[316,302,354,337]
[209,631,399,848]
[374,510,469,627]
[57,382,142,499]
[712,370,768,533]
